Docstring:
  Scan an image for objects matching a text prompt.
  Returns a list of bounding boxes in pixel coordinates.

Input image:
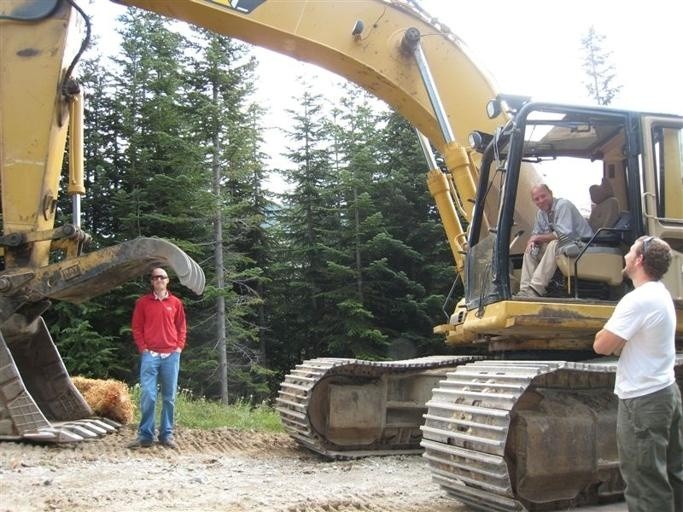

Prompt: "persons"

[126,267,187,448]
[593,232,683,511]
[514,183,594,297]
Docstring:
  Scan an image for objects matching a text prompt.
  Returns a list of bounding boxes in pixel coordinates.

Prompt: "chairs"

[556,177,635,302]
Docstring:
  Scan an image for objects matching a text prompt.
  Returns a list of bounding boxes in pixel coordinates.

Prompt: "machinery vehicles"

[2,0,682,510]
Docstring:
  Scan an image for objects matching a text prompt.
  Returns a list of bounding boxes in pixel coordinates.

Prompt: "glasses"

[643,237,654,251]
[151,274,168,281]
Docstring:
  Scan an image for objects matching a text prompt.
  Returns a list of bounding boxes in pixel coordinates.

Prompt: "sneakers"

[516,285,540,297]
[158,438,178,449]
[126,437,153,448]
